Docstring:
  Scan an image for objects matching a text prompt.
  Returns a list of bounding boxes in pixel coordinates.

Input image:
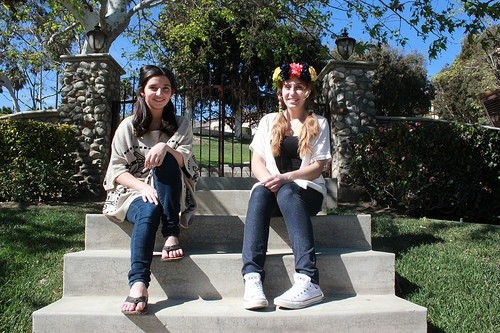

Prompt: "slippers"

[121,295,148,315]
[161,244,184,261]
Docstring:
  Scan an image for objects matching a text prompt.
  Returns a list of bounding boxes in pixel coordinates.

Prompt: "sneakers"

[274,274,324,309]
[244,272,269,309]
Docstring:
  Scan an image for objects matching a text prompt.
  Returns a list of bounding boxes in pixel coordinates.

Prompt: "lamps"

[87,22,107,53]
[335,26,356,62]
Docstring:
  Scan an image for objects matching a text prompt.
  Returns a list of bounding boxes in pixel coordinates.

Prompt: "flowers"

[272,63,317,90]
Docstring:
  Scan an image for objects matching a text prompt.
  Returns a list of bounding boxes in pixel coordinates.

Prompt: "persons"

[103,64,200,317]
[238,60,336,308]
